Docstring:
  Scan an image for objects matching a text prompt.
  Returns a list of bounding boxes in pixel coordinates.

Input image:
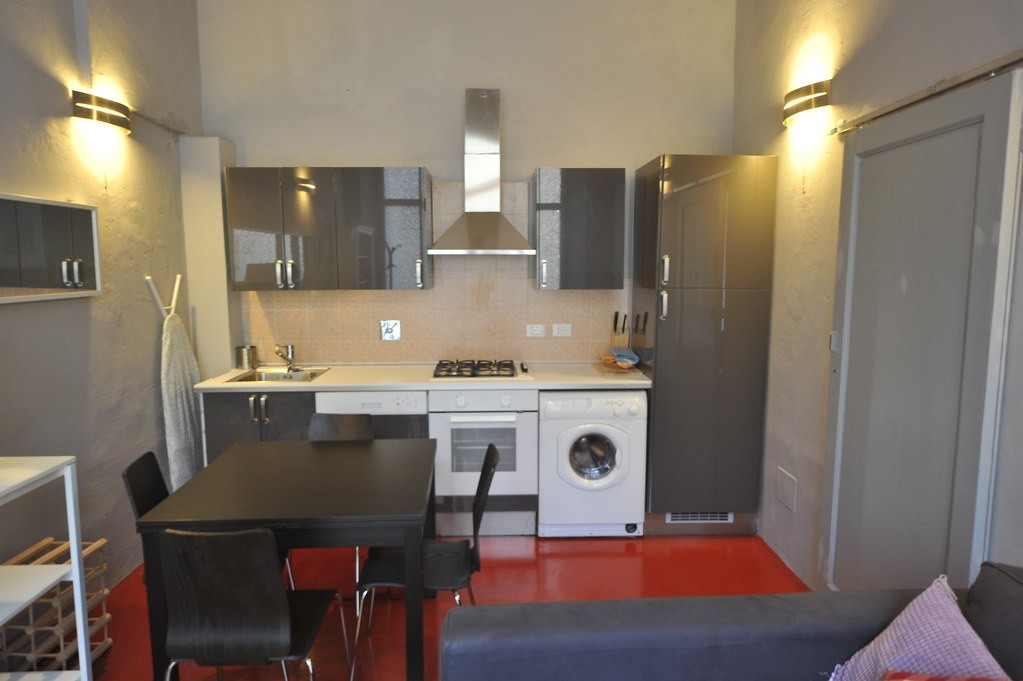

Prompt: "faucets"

[274,343,300,372]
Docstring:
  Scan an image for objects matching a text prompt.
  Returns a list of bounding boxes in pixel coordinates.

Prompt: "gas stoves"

[433,359,516,377]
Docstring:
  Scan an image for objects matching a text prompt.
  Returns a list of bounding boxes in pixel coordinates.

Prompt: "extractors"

[426,86,538,255]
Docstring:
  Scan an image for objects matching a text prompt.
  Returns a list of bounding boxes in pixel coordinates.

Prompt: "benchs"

[536,167,625,290]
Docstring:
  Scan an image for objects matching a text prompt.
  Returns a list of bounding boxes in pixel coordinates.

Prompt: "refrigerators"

[629,153,768,540]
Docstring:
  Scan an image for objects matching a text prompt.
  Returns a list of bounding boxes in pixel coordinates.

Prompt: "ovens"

[425,389,538,537]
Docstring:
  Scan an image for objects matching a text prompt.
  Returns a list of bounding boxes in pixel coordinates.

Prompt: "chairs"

[121,414,501,681]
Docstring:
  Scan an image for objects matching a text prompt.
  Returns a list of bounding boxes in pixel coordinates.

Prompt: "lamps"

[67,75,131,183]
[782,36,833,176]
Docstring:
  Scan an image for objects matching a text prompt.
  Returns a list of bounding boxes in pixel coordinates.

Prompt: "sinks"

[223,368,331,383]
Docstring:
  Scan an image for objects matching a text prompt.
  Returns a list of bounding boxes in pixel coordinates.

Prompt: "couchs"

[440,561,1023,681]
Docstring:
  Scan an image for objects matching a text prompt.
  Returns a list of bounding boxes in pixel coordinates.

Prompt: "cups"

[235,345,257,370]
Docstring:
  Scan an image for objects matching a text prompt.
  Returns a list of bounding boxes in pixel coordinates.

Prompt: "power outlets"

[552,324,572,337]
[527,324,546,338]
[380,320,400,341]
[773,466,797,511]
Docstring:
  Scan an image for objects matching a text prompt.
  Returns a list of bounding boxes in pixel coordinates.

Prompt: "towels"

[610,347,639,364]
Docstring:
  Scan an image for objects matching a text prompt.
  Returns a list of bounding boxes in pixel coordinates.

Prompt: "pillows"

[828,574,1012,681]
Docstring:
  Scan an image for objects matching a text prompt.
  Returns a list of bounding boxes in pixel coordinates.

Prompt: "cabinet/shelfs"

[203,391,435,539]
[334,167,435,290]
[0,192,103,304]
[0,538,116,672]
[0,455,96,681]
[225,166,338,292]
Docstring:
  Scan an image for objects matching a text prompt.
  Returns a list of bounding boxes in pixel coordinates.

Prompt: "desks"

[135,438,437,681]
